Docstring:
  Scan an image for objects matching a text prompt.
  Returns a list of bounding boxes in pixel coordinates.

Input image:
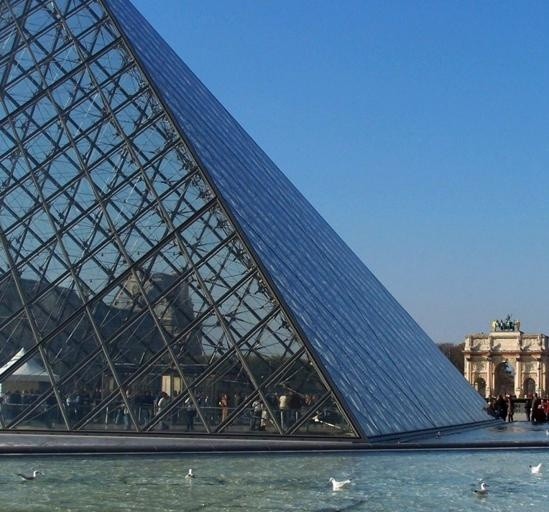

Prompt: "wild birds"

[328,477,351,491]
[529,463,544,476]
[185,469,195,479]
[472,482,490,493]
[14,470,41,481]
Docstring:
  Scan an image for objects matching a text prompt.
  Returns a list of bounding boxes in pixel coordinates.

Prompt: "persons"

[2,385,341,433]
[485,390,549,425]
